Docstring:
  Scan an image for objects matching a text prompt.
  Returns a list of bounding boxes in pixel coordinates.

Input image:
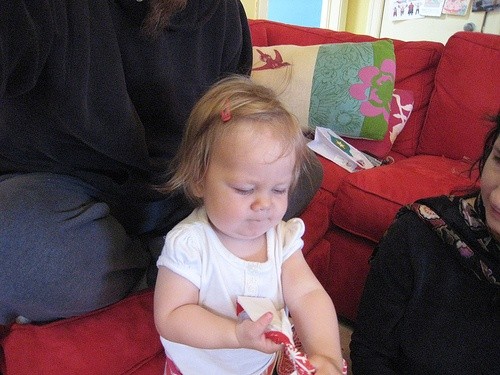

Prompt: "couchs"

[1,19,500,374]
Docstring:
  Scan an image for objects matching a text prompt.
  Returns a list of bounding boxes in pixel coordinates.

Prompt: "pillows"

[250,39,397,141]
[337,87,414,159]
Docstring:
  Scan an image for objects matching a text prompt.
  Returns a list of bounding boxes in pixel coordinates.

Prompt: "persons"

[0,0,253,319]
[152,78,342,375]
[349,113,500,375]
